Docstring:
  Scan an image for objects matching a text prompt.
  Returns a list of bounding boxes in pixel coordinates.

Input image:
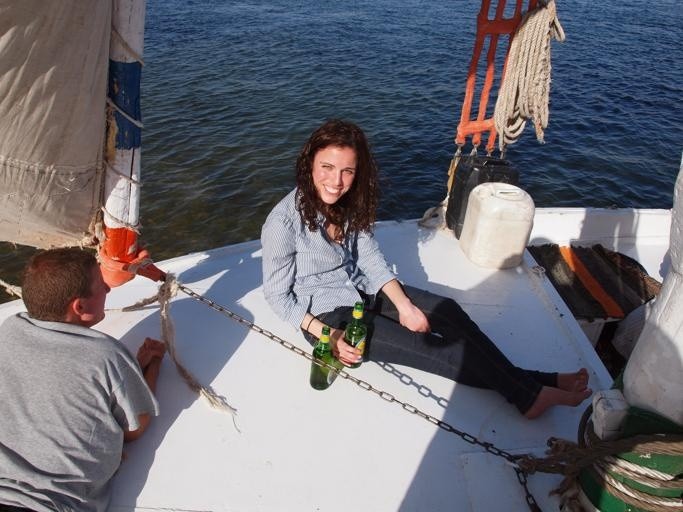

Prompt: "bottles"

[344,302,368,368]
[310,326,333,390]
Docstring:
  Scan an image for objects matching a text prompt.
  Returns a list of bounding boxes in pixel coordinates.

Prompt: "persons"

[1,249,167,511]
[261,120,593,420]
